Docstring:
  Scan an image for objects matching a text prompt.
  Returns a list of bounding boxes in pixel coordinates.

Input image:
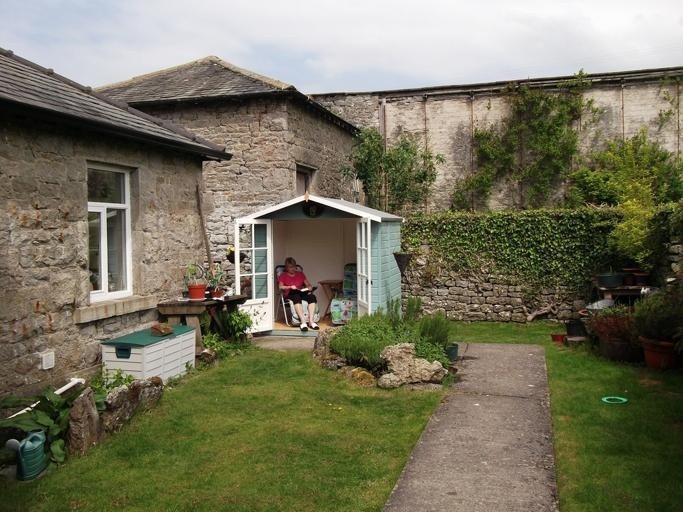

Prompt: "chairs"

[276,265,303,326]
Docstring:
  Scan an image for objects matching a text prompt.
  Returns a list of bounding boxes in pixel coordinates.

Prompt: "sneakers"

[300,323,319,331]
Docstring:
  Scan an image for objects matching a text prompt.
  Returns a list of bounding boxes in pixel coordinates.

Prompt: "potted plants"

[632,287,683,366]
[207,263,226,298]
[182,262,209,298]
[393,251,413,275]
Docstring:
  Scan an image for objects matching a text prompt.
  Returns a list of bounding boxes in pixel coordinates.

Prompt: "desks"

[318,279,343,321]
[157,294,248,356]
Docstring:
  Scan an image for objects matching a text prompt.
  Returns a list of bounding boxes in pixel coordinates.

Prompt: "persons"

[277,256,319,331]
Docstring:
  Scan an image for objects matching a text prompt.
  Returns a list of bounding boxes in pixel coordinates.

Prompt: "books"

[300,288,309,292]
